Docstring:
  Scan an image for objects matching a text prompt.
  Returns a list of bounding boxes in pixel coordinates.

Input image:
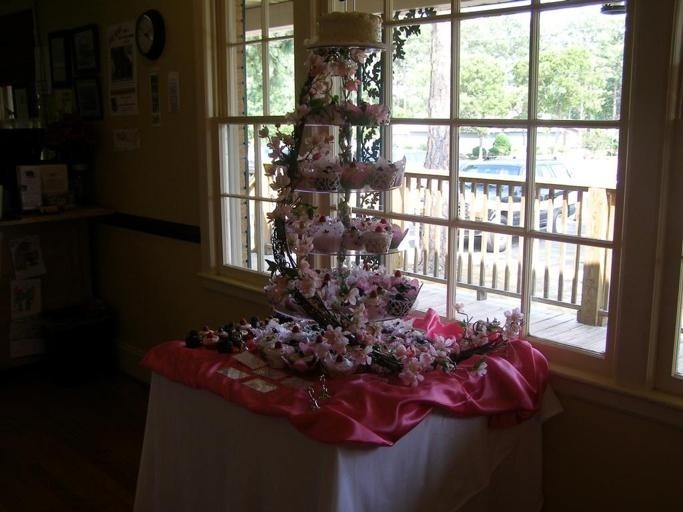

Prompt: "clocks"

[134,9,166,60]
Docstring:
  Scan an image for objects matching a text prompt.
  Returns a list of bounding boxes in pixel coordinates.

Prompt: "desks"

[1,206,116,370]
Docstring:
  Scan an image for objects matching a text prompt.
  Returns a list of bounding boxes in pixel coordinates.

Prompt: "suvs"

[406,155,584,256]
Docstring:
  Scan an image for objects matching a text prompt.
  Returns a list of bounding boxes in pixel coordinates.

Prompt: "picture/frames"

[47,24,104,121]
